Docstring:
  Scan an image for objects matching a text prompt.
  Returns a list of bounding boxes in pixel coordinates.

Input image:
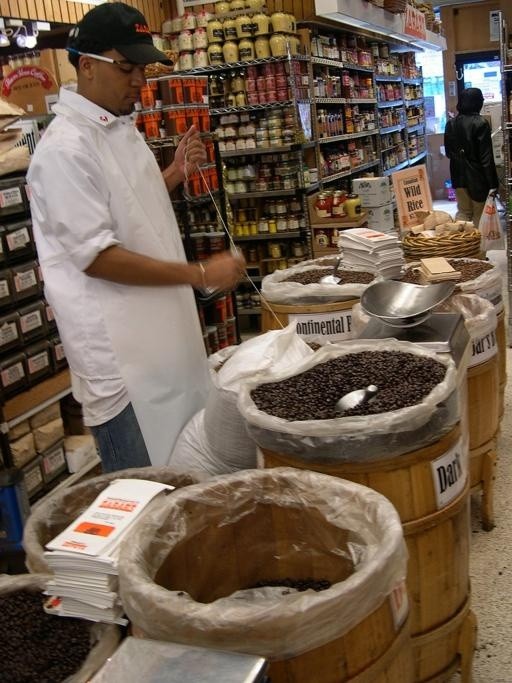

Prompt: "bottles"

[329,228,340,248]
[310,69,374,98]
[314,229,329,248]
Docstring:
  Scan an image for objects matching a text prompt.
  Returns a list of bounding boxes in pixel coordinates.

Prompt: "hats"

[65,2,174,69]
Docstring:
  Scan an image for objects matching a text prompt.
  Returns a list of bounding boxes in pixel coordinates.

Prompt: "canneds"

[193,49,208,67]
[171,16,181,31]
[315,189,362,218]
[261,0,267,8]
[222,40,239,61]
[196,9,208,28]
[178,30,193,51]
[302,136,376,187]
[295,36,301,55]
[205,61,308,106]
[222,17,237,40]
[206,18,223,42]
[152,34,163,51]
[245,0,261,9]
[270,10,297,33]
[214,108,296,152]
[222,153,298,193]
[169,35,178,50]
[178,231,237,356]
[235,13,252,38]
[228,197,305,236]
[254,36,271,58]
[179,52,193,69]
[214,0,229,14]
[238,241,308,274]
[229,0,244,12]
[269,32,287,56]
[310,33,400,76]
[161,19,171,32]
[238,38,255,61]
[290,35,296,56]
[252,11,268,35]
[192,28,208,49]
[181,13,196,29]
[207,43,223,65]
[134,74,220,199]
[316,105,375,138]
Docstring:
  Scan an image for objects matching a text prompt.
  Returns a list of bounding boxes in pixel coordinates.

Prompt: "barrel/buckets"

[359,295,506,530]
[25,464,201,581]
[260,268,381,344]
[394,258,506,423]
[132,466,412,683]
[242,341,477,683]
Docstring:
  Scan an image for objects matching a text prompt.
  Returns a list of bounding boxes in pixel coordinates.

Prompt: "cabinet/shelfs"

[302,25,430,230]
[140,72,247,352]
[176,16,319,301]
[4,162,103,500]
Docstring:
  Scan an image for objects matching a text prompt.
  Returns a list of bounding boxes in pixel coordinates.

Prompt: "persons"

[24,2,246,472]
[445,88,499,226]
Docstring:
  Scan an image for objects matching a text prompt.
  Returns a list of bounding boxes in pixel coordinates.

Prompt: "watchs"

[199,264,216,299]
